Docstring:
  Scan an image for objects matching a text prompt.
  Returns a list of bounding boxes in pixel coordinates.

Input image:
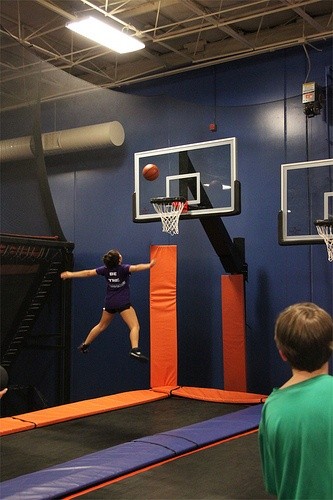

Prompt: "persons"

[256,303,333,499]
[60,250,156,361]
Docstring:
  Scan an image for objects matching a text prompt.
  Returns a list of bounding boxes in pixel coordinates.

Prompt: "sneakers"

[130,349,149,362]
[77,343,91,355]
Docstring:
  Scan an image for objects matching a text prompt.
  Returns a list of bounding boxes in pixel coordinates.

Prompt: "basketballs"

[142,163,160,181]
